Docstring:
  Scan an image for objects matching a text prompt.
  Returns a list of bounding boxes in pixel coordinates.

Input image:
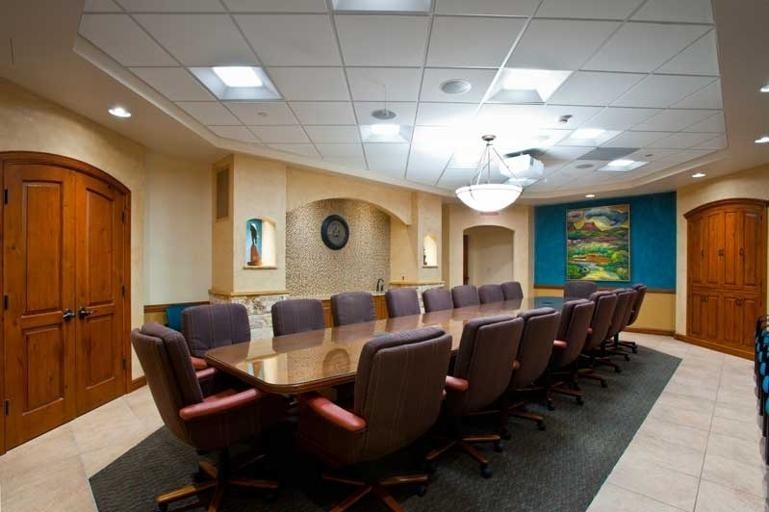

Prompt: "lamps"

[451,132,524,214]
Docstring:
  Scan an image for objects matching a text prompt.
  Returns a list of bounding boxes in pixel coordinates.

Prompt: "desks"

[203,297,554,389]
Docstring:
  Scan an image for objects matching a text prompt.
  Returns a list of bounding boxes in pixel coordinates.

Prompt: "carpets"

[87,341,684,511]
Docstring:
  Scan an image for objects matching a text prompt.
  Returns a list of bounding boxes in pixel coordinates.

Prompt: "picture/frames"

[564,202,632,283]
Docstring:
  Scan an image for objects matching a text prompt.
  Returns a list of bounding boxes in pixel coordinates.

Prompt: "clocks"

[321,214,350,249]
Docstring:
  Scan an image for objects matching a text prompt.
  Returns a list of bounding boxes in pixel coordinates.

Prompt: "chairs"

[178,302,253,354]
[502,281,524,300]
[562,282,597,298]
[454,316,545,442]
[295,326,470,511]
[583,284,647,374]
[331,289,376,327]
[550,298,609,392]
[513,304,586,412]
[127,321,296,509]
[421,287,455,312]
[451,285,480,308]
[270,297,325,339]
[477,285,506,304]
[385,287,422,318]
